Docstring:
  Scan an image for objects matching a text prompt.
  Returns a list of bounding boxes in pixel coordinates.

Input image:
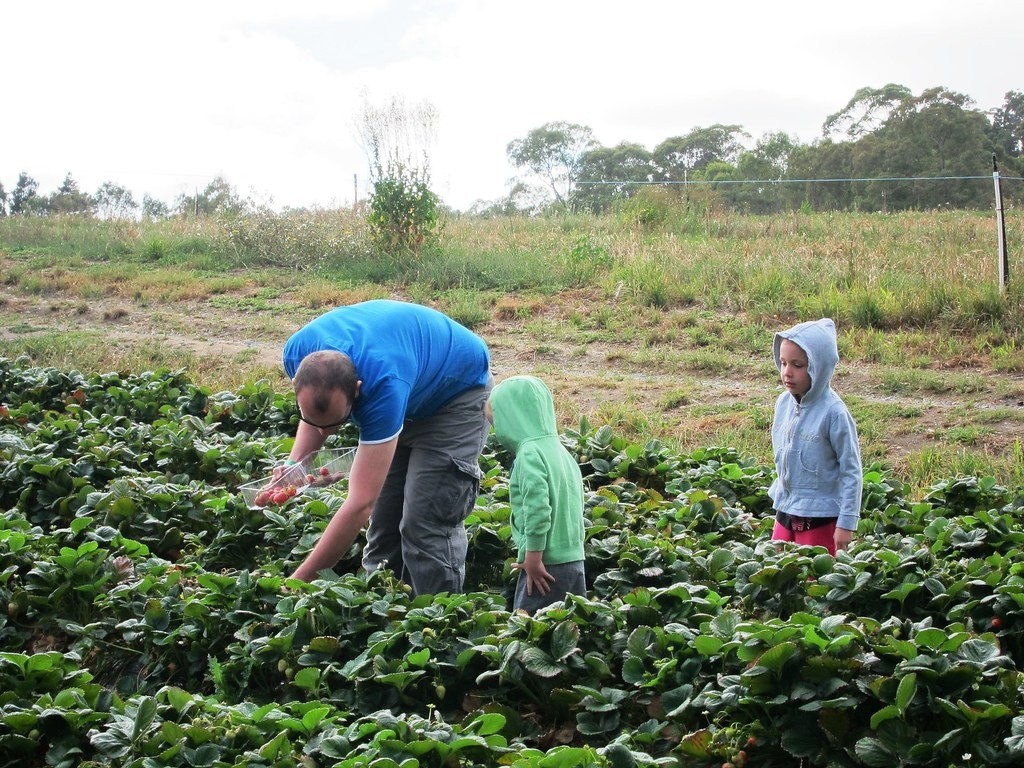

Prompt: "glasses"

[295,399,355,429]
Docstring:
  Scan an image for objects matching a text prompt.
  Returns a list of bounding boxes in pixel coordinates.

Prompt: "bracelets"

[285,460,296,465]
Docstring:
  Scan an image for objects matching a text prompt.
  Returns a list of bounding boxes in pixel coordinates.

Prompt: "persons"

[266,300,494,603]
[769,318,862,580]
[483,374,586,614]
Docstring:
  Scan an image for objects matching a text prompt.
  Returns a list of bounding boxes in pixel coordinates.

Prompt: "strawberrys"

[255,466,328,507]
